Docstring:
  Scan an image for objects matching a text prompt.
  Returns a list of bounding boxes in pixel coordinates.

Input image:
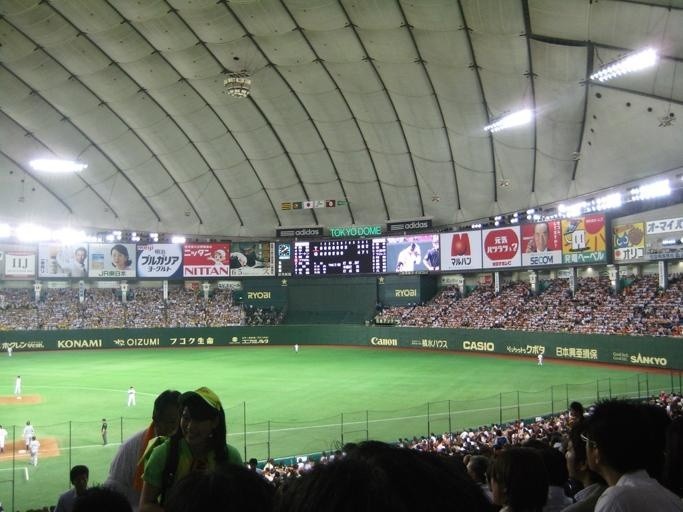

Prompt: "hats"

[179,386,222,410]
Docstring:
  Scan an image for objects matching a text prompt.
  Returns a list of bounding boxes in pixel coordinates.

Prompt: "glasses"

[580,430,598,446]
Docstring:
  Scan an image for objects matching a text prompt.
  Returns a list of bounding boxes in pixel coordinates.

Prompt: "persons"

[368,269,682,339]
[537,352,544,368]
[7,345,12,357]
[13,375,21,400]
[1,385,682,510]
[109,244,134,271]
[229,243,269,268]
[421,235,439,271]
[395,242,421,272]
[294,343,299,353]
[56,247,87,278]
[0,286,284,332]
[524,221,553,253]
